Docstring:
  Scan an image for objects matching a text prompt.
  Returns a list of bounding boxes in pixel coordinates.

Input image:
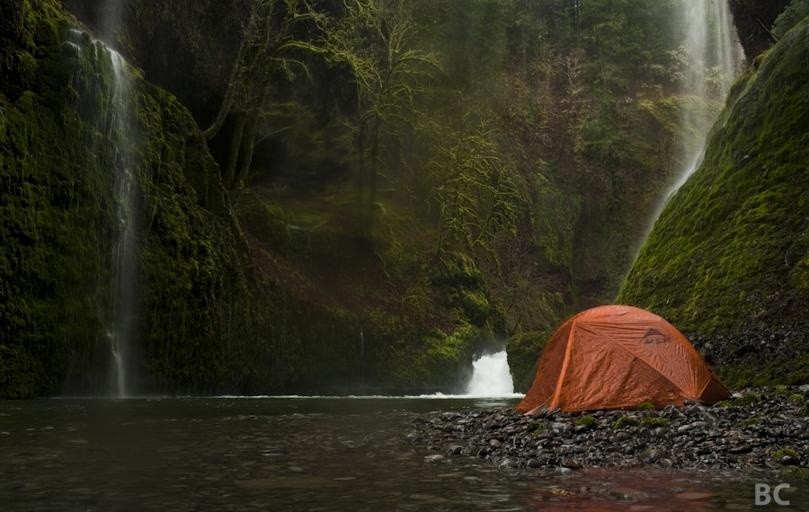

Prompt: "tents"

[516,305,732,410]
